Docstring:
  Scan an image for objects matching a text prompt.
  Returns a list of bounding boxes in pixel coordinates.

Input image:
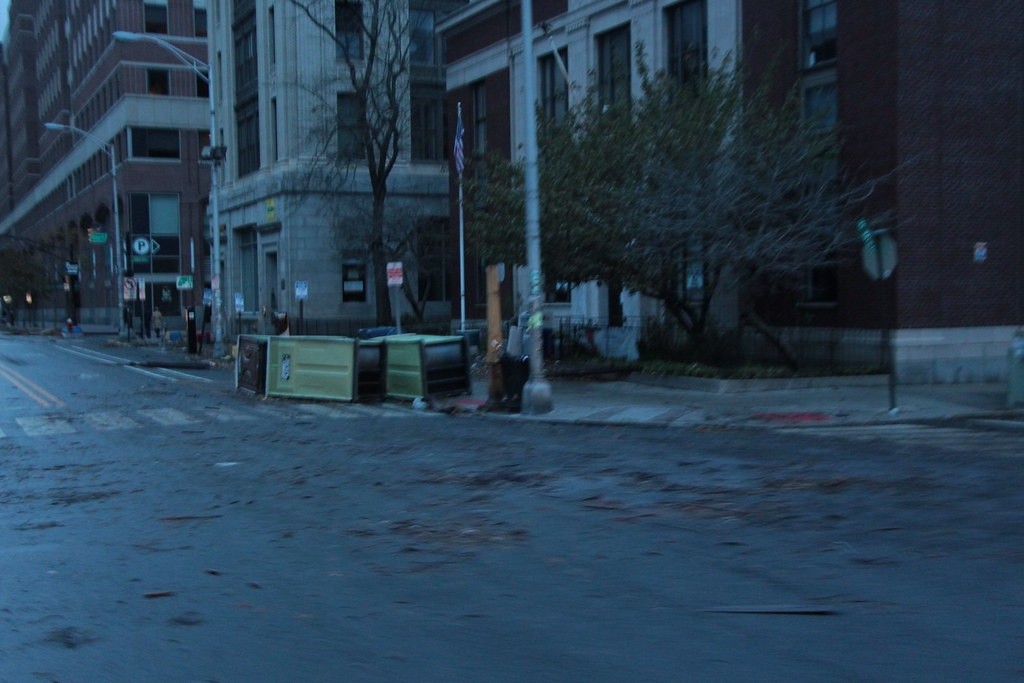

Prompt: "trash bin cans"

[500,352,529,409]
[456,329,481,349]
[359,326,405,338]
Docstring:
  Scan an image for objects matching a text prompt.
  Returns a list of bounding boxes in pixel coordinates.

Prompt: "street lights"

[43,122,128,339]
[112,30,226,357]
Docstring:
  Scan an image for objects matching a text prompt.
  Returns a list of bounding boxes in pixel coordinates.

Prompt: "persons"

[144,306,152,339]
[152,307,163,338]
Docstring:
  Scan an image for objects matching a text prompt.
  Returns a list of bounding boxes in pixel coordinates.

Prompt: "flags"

[452,105,468,178]
